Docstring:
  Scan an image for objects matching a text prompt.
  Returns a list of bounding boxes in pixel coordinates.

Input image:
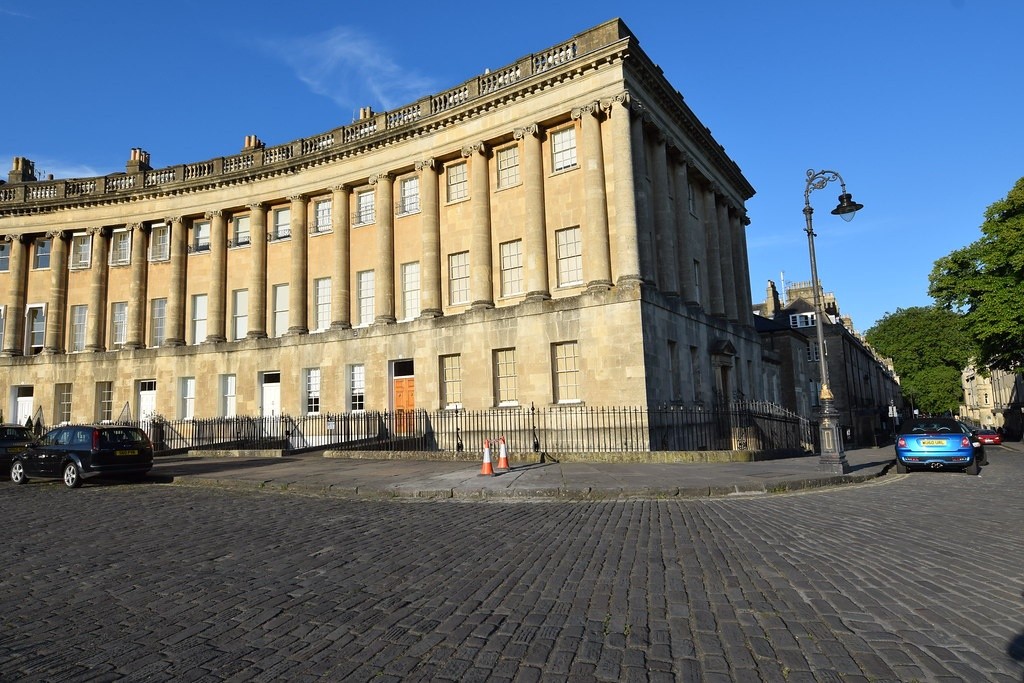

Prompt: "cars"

[957,422,987,466]
[895,417,978,476]
[975,429,1001,445]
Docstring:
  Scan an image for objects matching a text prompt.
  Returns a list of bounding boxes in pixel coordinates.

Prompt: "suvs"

[8,425,154,489]
[0,423,38,474]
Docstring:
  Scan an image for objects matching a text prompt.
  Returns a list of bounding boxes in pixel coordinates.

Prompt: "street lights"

[804,168,864,474]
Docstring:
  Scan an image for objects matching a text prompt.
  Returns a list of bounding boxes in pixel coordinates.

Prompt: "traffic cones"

[495,436,512,471]
[476,440,497,478]
